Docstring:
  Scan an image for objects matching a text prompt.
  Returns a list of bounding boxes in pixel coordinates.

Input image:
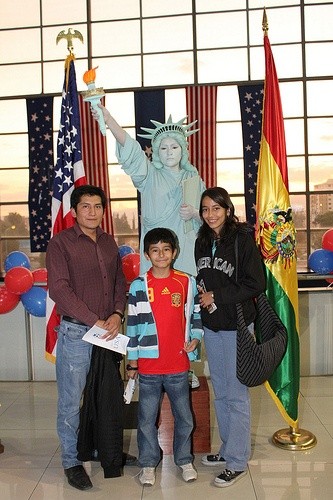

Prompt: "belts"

[62,315,87,325]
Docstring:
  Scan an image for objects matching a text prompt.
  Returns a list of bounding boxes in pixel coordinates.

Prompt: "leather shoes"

[64,464,93,490]
[120,452,137,465]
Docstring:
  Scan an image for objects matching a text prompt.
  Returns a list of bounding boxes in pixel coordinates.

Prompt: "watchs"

[126,364,138,371]
[112,312,125,325]
[211,291,214,299]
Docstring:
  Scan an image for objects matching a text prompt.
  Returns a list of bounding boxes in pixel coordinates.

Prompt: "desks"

[204,288,333,377]
[0,282,129,382]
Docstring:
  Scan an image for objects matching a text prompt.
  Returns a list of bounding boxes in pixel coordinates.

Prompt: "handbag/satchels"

[236,291,288,387]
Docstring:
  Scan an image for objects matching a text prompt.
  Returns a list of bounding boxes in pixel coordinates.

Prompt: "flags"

[253,31,302,433]
[43,54,89,364]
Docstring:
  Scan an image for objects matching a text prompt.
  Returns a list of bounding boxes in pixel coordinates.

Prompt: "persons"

[125,228,204,486]
[194,187,268,487]
[45,184,138,490]
[91,104,206,390]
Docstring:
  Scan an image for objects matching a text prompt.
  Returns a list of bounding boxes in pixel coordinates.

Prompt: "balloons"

[118,245,141,281]
[307,228,333,284]
[0,251,48,318]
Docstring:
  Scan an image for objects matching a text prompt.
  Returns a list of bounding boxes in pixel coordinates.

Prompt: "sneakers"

[201,454,227,466]
[179,463,197,482]
[214,467,247,488]
[133,467,155,486]
